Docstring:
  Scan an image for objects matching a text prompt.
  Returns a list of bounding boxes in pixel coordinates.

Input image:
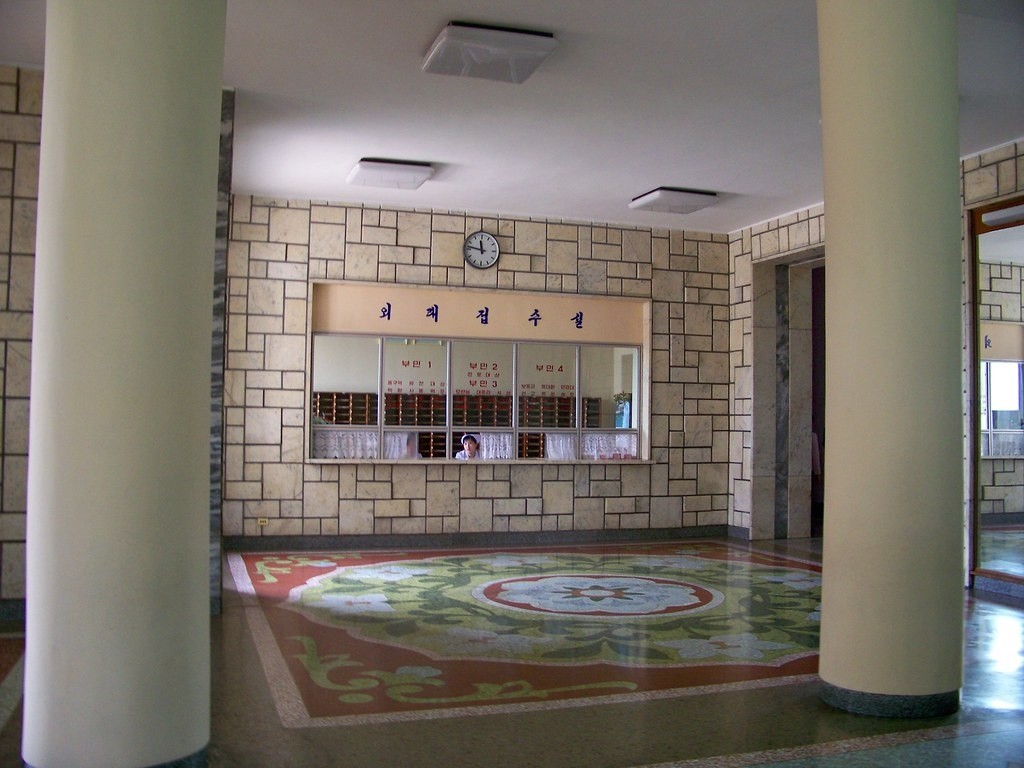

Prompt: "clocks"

[463,231,500,270]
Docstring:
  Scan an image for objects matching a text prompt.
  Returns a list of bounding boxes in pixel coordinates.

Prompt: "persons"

[455,433,481,458]
[400,434,422,458]
[983,431,1000,455]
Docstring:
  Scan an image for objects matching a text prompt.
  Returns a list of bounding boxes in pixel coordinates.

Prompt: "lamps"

[346,157,435,190]
[628,186,720,214]
[421,21,556,85]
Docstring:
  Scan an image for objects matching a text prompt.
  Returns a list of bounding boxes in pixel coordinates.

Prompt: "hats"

[460,434,481,444]
